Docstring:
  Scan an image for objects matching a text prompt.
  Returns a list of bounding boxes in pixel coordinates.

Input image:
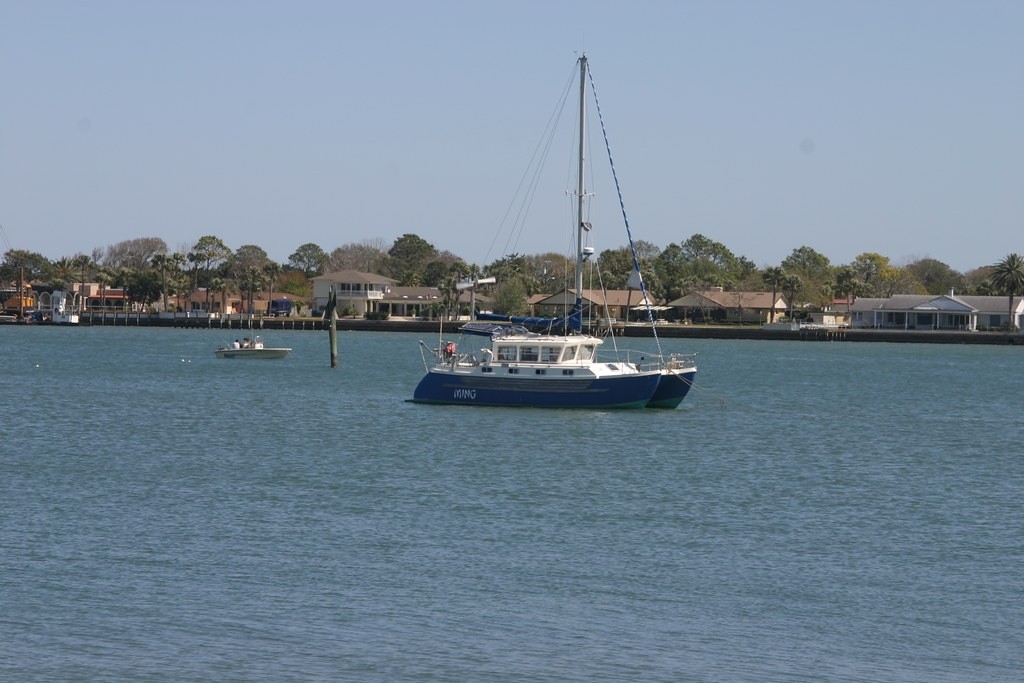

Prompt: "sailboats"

[401,50,701,412]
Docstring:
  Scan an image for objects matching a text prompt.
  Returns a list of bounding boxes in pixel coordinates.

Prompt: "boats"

[213,332,294,362]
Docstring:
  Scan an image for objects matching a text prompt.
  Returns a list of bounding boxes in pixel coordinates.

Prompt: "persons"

[232,336,255,348]
